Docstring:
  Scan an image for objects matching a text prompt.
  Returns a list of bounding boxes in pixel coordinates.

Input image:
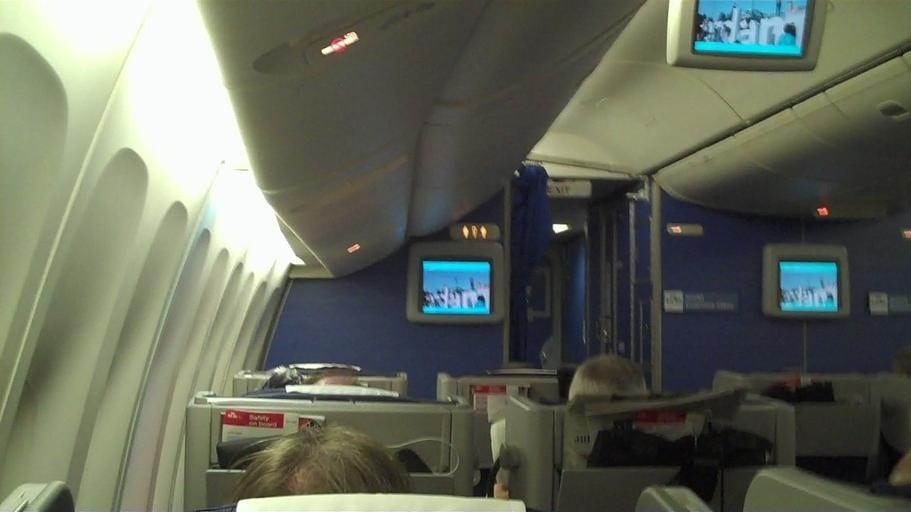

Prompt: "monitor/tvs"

[763,242,851,318]
[405,239,505,325]
[666,0,826,73]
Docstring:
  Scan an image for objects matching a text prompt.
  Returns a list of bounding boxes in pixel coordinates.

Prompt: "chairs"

[2,360,911,512]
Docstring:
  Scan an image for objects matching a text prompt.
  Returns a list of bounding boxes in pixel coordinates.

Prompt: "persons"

[566,354,648,404]
[227,421,410,502]
